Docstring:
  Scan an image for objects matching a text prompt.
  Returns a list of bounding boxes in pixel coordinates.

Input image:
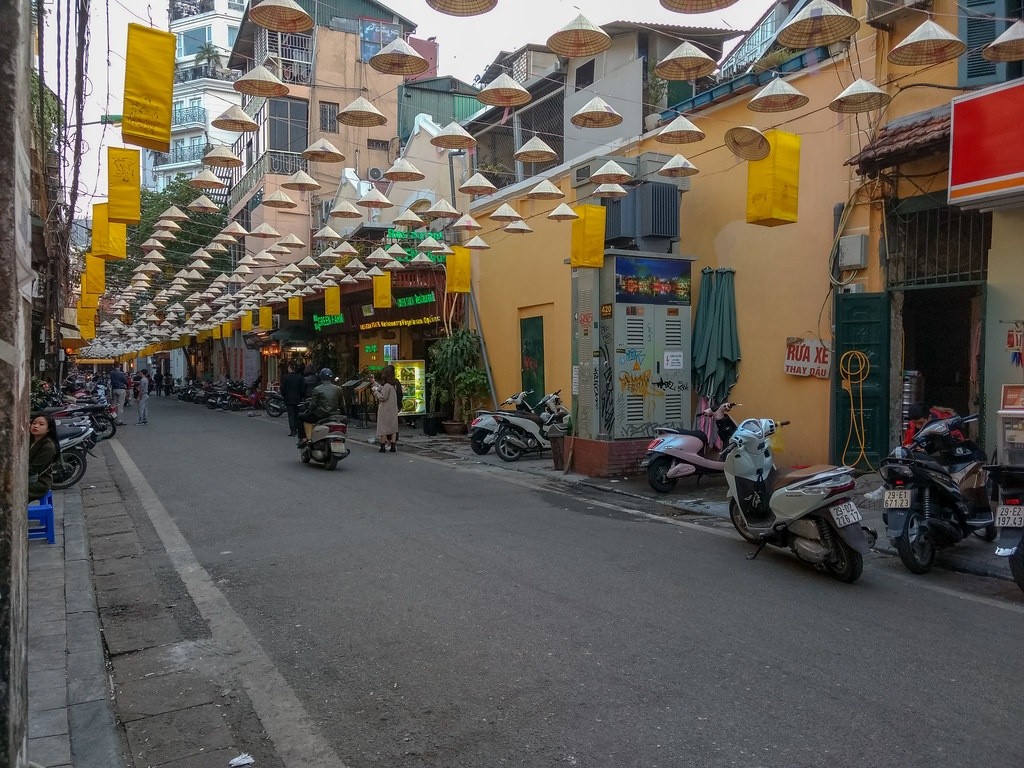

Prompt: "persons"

[251,375,263,409]
[371,366,403,453]
[94,361,169,425]
[61,386,76,403]
[281,365,320,436]
[903,405,964,452]
[296,368,347,449]
[28,414,60,503]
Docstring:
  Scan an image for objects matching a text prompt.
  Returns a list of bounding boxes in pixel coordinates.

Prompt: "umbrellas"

[692,266,741,453]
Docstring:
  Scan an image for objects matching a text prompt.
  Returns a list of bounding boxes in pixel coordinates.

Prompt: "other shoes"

[288,430,297,436]
[297,440,307,448]
[116,421,127,425]
[136,421,144,425]
[144,421,148,425]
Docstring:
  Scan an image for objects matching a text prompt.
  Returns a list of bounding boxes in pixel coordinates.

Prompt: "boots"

[389,441,396,452]
[379,443,385,452]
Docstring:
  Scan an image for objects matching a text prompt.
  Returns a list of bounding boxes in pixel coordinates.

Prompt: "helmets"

[320,368,332,380]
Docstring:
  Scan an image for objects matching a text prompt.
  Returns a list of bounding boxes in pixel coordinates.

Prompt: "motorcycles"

[641,400,743,493]
[881,418,997,576]
[148,382,172,397]
[983,464,1024,589]
[177,377,290,417]
[493,389,572,462]
[298,401,350,472]
[720,420,878,584]
[132,381,142,400]
[29,382,129,489]
[468,390,535,456]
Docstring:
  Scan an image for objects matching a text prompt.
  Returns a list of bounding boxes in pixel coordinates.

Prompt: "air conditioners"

[367,167,389,182]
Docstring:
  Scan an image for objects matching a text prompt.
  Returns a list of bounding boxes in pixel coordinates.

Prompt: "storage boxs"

[660,47,829,118]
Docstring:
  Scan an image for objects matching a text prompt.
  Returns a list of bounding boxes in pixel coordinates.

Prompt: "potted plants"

[454,366,489,436]
[642,56,663,131]
[351,400,378,422]
[422,326,484,436]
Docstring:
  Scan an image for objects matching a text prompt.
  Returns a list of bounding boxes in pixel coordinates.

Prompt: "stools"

[26,504,56,544]
[32,488,53,525]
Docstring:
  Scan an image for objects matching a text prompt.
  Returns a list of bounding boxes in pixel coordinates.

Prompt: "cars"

[130,373,139,380]
